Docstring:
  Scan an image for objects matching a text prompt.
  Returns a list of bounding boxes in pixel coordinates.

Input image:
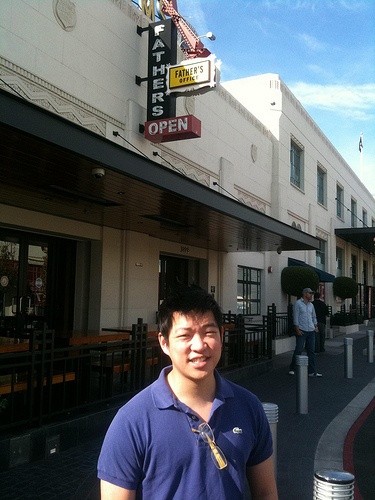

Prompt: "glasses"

[192,423,228,471]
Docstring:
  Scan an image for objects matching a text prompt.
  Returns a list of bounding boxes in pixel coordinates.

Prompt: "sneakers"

[309,373,322,377]
[289,371,294,374]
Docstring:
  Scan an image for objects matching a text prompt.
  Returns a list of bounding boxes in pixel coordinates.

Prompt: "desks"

[0,322,235,394]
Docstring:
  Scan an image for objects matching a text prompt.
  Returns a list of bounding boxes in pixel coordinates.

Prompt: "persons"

[97,285,278,500]
[311,292,330,352]
[288,287,323,378]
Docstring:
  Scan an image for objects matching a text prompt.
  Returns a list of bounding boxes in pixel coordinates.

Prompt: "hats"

[303,288,315,292]
